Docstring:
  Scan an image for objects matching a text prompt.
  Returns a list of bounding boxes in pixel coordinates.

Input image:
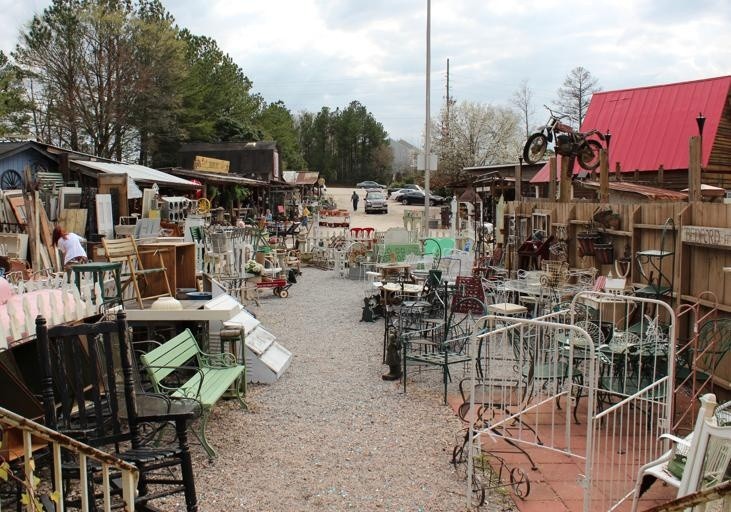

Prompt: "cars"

[357,181,386,189]
[388,184,444,206]
[364,189,387,214]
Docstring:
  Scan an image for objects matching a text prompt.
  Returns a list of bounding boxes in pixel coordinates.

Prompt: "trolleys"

[257,280,292,298]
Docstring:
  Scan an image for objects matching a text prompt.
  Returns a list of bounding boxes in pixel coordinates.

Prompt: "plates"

[185,292,212,300]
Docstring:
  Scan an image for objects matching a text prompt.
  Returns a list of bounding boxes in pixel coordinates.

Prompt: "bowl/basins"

[176,287,197,300]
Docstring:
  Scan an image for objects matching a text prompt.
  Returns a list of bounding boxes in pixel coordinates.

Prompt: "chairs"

[35,235,204,512]
[359,242,731,512]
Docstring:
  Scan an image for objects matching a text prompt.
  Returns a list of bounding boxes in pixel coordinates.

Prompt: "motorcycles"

[523,104,605,170]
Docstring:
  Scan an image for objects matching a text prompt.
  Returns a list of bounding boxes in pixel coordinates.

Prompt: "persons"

[50,225,90,273]
[260,207,267,222]
[300,203,311,230]
[264,208,273,222]
[350,190,360,212]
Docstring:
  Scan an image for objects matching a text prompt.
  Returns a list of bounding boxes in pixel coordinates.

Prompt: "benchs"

[140,328,248,459]
